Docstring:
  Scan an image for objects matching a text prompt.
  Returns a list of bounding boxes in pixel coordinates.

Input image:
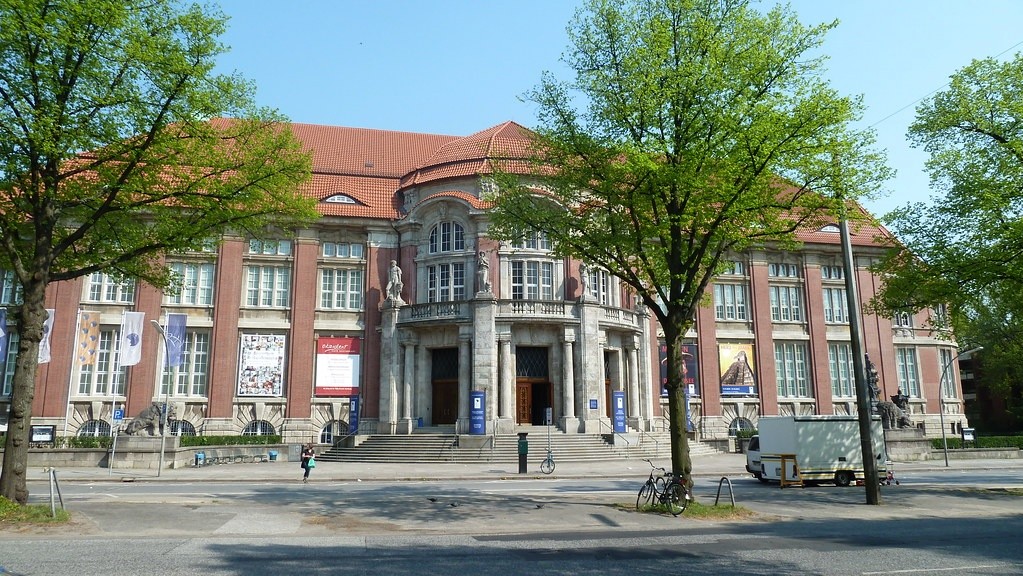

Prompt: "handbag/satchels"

[308,458,315,468]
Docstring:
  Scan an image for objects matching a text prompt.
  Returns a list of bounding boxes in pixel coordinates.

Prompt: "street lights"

[150,319,171,477]
[938,345,984,467]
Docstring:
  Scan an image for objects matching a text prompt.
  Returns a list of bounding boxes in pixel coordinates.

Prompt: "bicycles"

[635,458,691,516]
[540,447,556,474]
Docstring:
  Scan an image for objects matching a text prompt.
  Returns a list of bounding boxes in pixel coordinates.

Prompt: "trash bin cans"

[415,417,424,427]
[270,451,277,460]
[195,453,205,465]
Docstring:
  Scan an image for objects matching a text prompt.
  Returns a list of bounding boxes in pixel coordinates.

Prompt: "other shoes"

[303,477,307,483]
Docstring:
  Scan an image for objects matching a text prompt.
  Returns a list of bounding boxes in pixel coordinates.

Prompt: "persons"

[870,362,881,395]
[638,277,650,313]
[384,260,402,301]
[477,251,489,292]
[579,262,591,294]
[302,445,315,483]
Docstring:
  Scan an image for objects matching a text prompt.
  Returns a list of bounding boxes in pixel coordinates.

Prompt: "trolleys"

[885,464,900,486]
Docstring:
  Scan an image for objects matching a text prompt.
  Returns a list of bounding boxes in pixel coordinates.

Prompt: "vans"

[745,434,772,483]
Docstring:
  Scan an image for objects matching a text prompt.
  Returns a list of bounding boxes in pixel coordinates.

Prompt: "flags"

[37,310,54,364]
[76,311,100,365]
[165,315,187,366]
[120,312,145,366]
[0,309,7,363]
